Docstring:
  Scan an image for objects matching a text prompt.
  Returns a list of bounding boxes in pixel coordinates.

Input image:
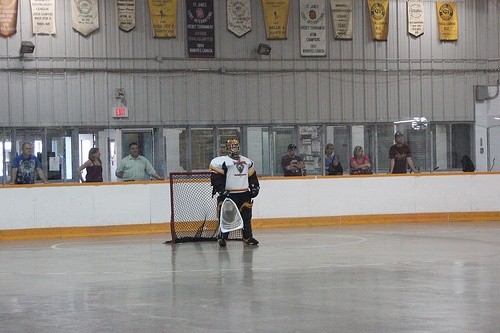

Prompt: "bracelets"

[43,180,46,183]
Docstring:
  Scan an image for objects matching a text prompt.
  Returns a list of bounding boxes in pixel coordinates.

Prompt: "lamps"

[17,40,35,60]
[256,43,272,60]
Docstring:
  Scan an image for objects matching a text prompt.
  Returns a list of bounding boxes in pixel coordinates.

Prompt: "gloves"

[250,190,257,197]
[222,191,230,198]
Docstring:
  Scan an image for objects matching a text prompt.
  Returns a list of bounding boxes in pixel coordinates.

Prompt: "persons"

[77,148,103,183]
[115,143,164,182]
[317,143,334,176]
[208,138,261,246]
[328,155,343,176]
[388,131,418,173]
[349,146,373,174]
[282,143,304,176]
[10,141,49,184]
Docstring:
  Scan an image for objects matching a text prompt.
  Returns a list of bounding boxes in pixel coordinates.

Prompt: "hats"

[396,131,403,136]
[288,144,296,149]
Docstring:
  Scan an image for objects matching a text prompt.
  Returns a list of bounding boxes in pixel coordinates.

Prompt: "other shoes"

[244,238,259,245]
[218,238,226,247]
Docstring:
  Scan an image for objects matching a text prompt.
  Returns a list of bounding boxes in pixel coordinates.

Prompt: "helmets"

[226,139,240,154]
